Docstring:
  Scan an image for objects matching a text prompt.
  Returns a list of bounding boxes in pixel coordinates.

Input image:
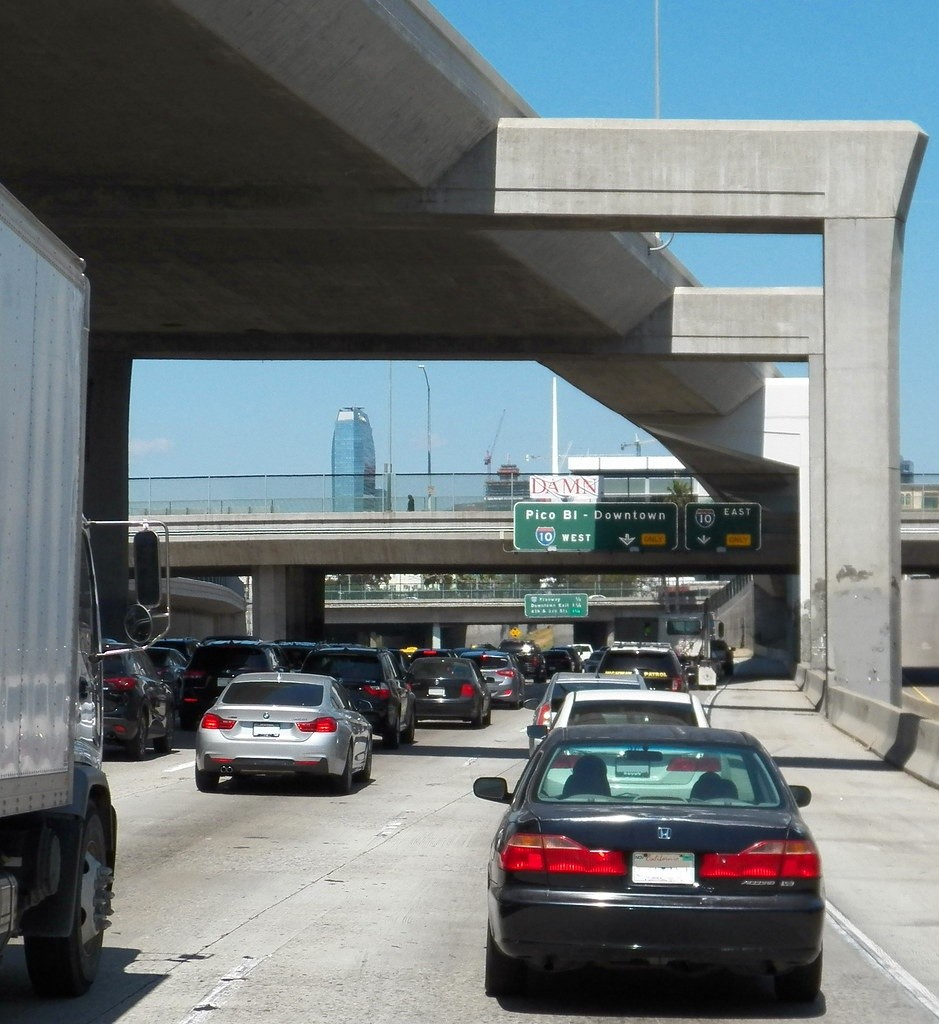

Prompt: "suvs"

[102,637,416,761]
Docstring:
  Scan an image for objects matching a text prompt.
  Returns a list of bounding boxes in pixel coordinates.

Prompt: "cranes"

[483,408,506,477]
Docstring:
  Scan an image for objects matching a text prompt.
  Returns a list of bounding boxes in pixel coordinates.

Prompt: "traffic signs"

[684,502,762,552]
[514,502,678,556]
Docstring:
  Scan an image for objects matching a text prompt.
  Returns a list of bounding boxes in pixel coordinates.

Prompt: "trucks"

[1,218,170,999]
[656,611,724,678]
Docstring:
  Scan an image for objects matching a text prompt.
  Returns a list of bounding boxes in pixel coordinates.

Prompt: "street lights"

[418,365,432,511]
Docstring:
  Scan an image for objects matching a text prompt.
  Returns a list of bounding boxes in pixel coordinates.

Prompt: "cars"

[711,638,736,677]
[529,639,698,761]
[194,672,373,794]
[526,689,731,800]
[405,640,593,729]
[473,725,825,1002]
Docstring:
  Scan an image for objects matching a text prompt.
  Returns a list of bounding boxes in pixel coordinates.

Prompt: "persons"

[573,755,606,788]
[408,494,414,510]
